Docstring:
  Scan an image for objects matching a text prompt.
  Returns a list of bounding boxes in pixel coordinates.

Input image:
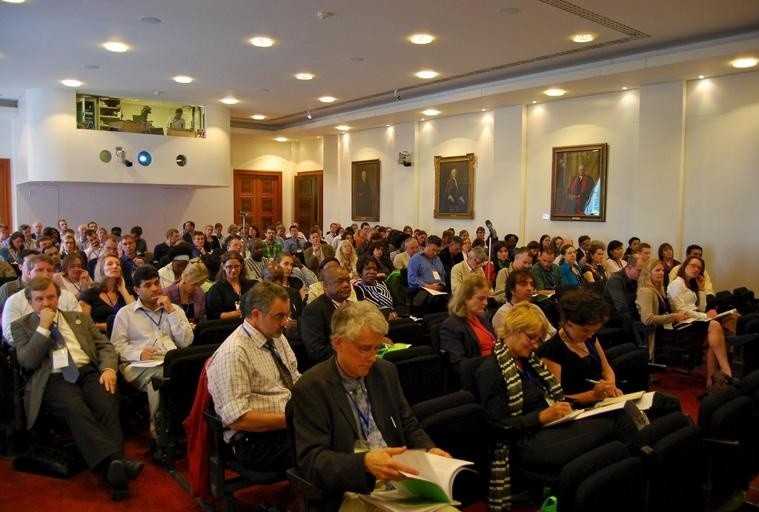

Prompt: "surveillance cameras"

[402,160,412,167]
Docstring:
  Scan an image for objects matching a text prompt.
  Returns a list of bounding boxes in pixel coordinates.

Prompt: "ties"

[50,324,80,384]
[264,341,293,387]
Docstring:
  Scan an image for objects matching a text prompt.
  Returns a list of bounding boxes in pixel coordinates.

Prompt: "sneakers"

[98,460,142,486]
[105,459,129,501]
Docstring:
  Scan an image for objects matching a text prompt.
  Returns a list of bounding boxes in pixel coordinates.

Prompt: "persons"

[560,164,595,216]
[0,216,759,510]
[358,170,372,217]
[167,108,185,130]
[445,169,467,213]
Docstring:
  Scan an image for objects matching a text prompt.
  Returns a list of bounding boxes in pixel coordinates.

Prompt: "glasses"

[223,265,242,270]
[347,337,385,355]
[521,328,545,346]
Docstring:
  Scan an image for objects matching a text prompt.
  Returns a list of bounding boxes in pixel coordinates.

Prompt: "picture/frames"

[549,142,608,222]
[433,153,475,220]
[351,159,381,222]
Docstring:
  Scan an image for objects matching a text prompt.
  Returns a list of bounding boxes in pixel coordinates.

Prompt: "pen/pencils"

[550,393,559,403]
[153,339,157,346]
[390,416,396,429]
[586,379,600,383]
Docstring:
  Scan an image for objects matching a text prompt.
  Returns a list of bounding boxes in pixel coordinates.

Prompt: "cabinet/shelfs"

[98,97,122,131]
